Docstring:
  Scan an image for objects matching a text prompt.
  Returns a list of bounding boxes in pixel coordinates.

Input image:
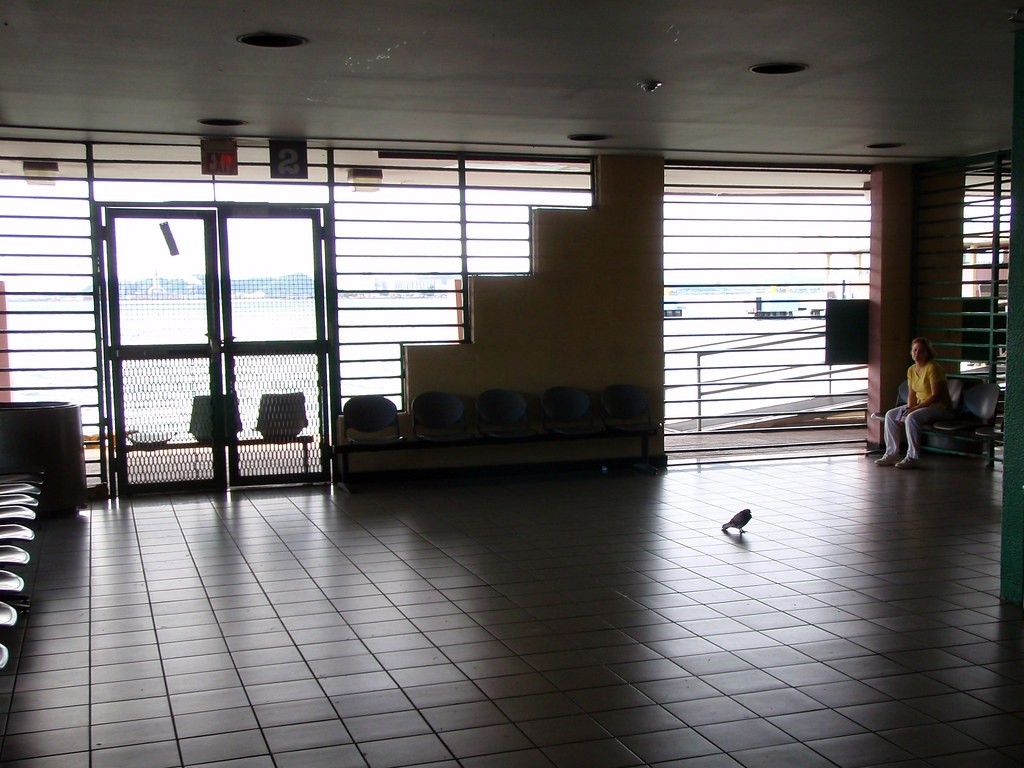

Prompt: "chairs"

[332,383,663,497]
[869,376,1004,468]
[0,469,47,670]
[126,395,314,477]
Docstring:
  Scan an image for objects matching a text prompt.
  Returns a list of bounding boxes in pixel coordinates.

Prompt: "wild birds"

[721,509,752,533]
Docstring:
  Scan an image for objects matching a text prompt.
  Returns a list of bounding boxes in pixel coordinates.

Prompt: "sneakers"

[874,454,901,466]
[895,457,918,469]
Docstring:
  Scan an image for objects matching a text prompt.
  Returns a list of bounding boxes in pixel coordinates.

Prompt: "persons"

[875,337,953,468]
